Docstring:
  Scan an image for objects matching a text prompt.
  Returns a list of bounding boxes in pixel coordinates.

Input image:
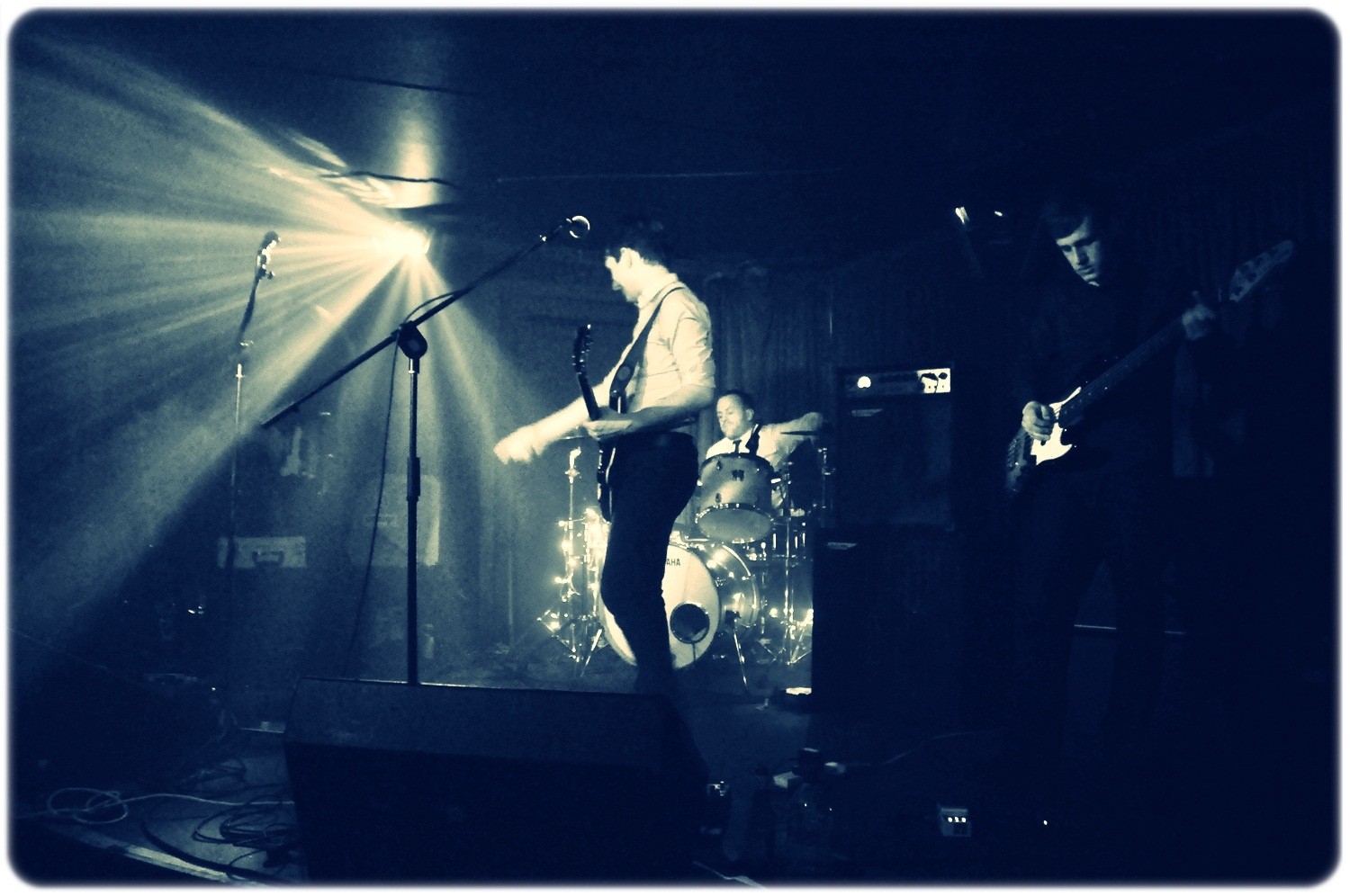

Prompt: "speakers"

[817,358,1009,737]
[8,628,232,819]
[285,677,709,887]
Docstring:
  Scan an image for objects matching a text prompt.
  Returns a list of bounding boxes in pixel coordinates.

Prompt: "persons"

[1012,198,1217,770]
[708,390,822,509]
[494,215,715,692]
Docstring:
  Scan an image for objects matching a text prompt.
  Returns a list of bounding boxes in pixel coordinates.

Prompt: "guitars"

[1003,233,1304,487]
[569,322,618,523]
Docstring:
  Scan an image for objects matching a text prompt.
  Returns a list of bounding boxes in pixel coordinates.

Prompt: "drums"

[697,451,777,545]
[670,491,694,533]
[744,520,808,567]
[595,538,760,671]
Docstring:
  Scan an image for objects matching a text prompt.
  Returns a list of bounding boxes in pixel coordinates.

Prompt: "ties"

[733,440,742,453]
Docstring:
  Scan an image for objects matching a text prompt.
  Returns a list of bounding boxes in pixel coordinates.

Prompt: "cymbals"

[780,429,836,437]
[545,432,593,450]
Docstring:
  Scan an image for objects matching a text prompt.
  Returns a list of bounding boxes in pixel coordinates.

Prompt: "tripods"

[504,443,604,678]
[754,473,812,665]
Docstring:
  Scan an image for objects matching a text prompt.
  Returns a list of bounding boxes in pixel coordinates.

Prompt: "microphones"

[565,215,590,238]
[258,230,280,255]
